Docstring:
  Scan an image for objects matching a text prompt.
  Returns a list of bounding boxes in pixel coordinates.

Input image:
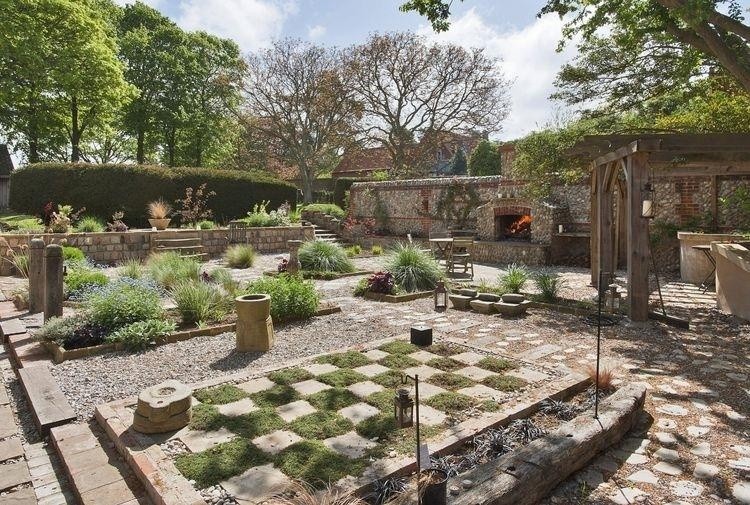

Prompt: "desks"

[429,237,466,267]
[692,244,721,295]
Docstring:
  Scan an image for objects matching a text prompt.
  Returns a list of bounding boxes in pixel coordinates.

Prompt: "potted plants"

[147,194,171,229]
[167,277,199,323]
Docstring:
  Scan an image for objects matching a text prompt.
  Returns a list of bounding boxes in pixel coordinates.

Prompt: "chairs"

[446,235,474,281]
